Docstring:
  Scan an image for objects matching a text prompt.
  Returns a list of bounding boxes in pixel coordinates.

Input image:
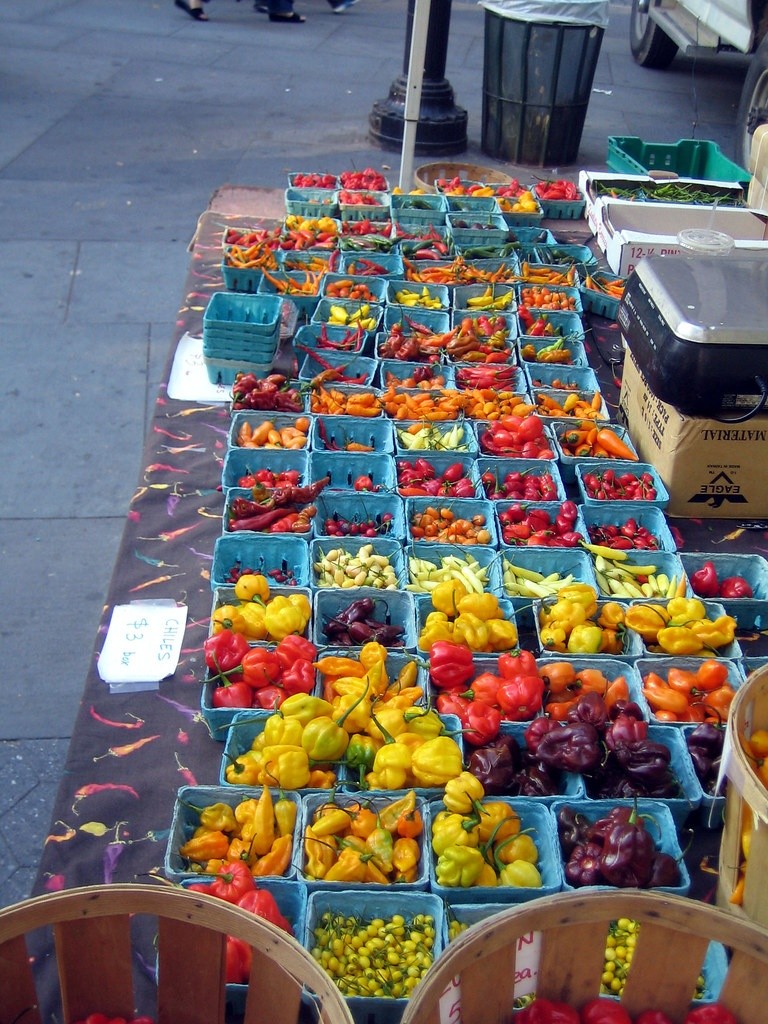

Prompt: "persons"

[175,0,307,24]
[253,0,360,14]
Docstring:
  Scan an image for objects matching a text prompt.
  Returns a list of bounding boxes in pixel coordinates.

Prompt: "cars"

[630,1,768,202]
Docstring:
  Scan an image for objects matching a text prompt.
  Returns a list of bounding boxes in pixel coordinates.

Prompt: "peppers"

[181,167,768,1024]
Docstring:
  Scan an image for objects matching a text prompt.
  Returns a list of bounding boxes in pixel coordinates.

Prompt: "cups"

[677,228,737,257]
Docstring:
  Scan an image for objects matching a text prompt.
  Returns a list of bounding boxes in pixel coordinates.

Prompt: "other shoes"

[254,4,268,13]
[334,0,357,13]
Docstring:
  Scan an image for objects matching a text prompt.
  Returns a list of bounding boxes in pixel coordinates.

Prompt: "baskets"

[163,172,768,1024]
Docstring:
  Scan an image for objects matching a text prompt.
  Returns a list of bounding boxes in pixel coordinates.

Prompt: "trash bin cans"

[477,0,610,169]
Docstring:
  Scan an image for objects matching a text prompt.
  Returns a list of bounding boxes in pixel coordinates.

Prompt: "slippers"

[175,0,209,22]
[269,12,306,22]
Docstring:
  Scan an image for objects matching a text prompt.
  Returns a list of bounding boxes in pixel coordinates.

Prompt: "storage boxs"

[156,137,768,1024]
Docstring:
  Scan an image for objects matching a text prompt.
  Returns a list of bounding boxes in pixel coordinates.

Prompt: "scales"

[614,255,768,424]
